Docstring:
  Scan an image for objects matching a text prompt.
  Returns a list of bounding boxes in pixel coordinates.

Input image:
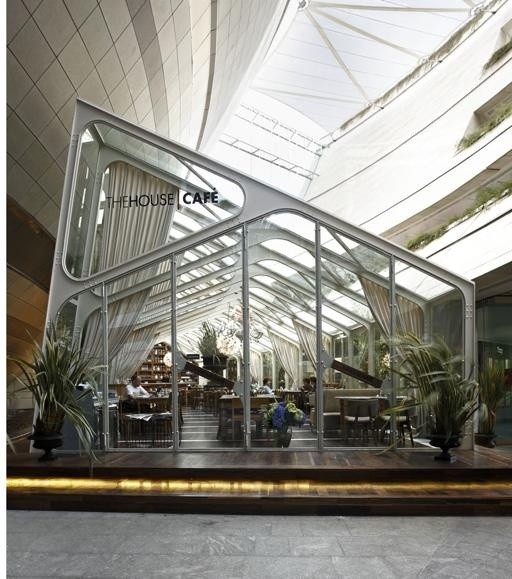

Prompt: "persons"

[306,377,317,401]
[263,380,273,395]
[303,377,310,389]
[120,374,149,411]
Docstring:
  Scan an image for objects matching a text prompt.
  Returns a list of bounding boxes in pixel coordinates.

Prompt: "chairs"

[89,379,418,451]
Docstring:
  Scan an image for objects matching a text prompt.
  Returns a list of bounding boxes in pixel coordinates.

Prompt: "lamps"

[213,300,241,355]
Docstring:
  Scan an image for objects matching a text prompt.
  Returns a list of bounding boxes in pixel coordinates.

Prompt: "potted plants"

[474,358,510,449]
[367,329,486,463]
[8,312,104,474]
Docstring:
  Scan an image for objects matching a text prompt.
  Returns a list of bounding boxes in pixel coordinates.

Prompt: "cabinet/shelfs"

[137,343,171,382]
